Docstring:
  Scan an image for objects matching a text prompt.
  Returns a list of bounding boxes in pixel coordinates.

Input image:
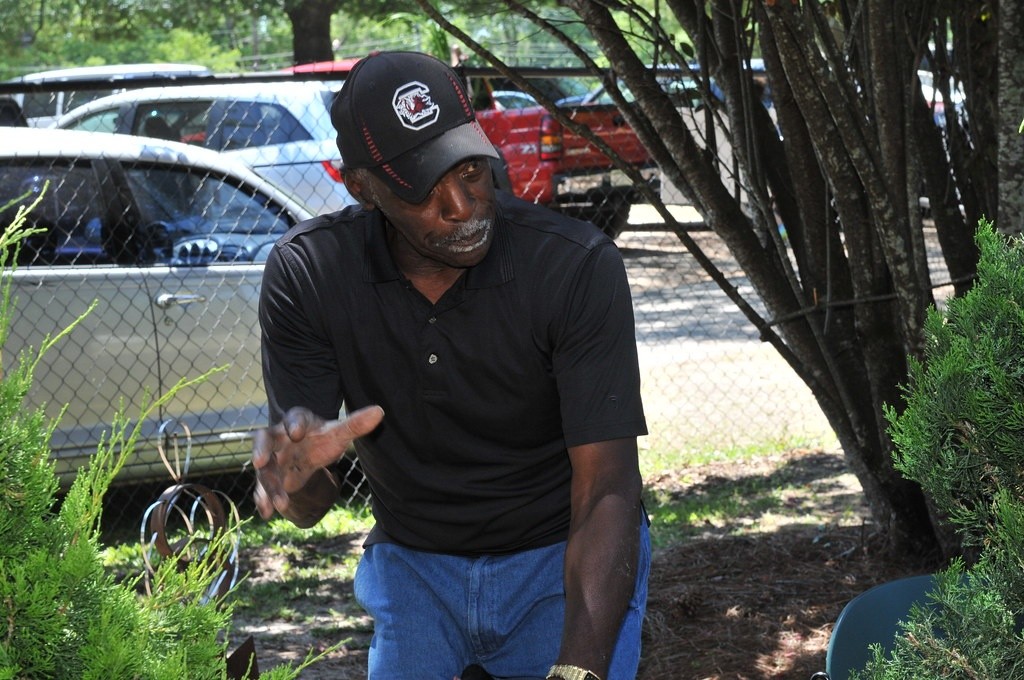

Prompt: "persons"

[247,47,653,680]
[449,44,471,68]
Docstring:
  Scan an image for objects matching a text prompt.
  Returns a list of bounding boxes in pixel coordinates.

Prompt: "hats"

[331,49,500,203]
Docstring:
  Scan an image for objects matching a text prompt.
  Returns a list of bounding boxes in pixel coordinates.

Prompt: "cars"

[2,63,210,136]
[46,78,358,217]
[555,42,967,217]
[1,125,357,493]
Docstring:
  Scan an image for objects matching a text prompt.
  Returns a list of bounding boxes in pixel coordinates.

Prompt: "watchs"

[546,665,600,680]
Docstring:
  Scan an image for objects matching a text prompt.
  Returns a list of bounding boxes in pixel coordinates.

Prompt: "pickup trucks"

[180,56,661,241]
